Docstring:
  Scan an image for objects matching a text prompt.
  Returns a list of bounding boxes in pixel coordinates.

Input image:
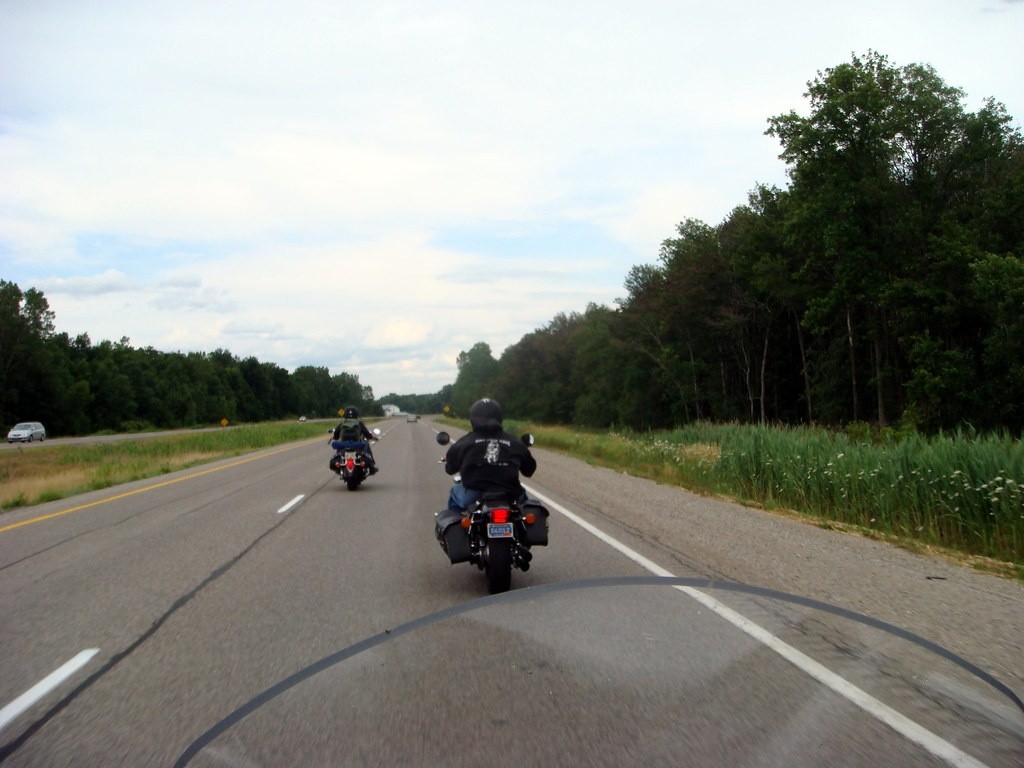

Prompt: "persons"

[328,406,380,475]
[443,397,536,510]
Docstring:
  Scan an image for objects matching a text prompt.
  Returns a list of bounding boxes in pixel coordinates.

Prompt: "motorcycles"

[328,428,381,492]
[434,432,550,596]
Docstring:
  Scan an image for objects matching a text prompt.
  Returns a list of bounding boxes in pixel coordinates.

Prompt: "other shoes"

[370,466,378,475]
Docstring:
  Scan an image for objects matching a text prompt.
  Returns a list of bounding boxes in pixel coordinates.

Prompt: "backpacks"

[342,421,360,441]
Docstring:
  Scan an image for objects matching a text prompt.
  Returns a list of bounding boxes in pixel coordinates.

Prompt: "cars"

[407,415,418,423]
[299,416,306,422]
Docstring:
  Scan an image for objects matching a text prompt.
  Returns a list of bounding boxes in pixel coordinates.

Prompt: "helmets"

[345,407,357,419]
[470,398,502,426]
